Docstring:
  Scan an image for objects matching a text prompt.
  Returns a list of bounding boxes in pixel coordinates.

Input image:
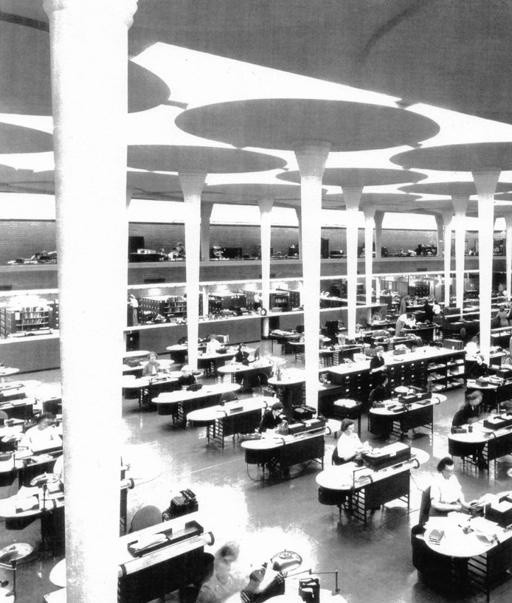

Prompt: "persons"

[127,292,139,326]
[230,341,251,384]
[141,350,167,378]
[19,411,63,453]
[336,416,373,467]
[370,373,393,407]
[252,290,262,314]
[390,290,443,340]
[206,334,222,354]
[259,402,285,435]
[194,544,248,602]
[178,364,198,391]
[449,280,512,438]
[428,455,475,519]
[369,344,387,399]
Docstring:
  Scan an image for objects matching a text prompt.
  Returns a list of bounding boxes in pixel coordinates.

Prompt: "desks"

[0,360,68,602]
[126,286,511,601]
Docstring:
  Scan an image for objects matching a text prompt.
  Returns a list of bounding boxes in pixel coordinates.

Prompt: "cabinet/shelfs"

[0,299,60,338]
